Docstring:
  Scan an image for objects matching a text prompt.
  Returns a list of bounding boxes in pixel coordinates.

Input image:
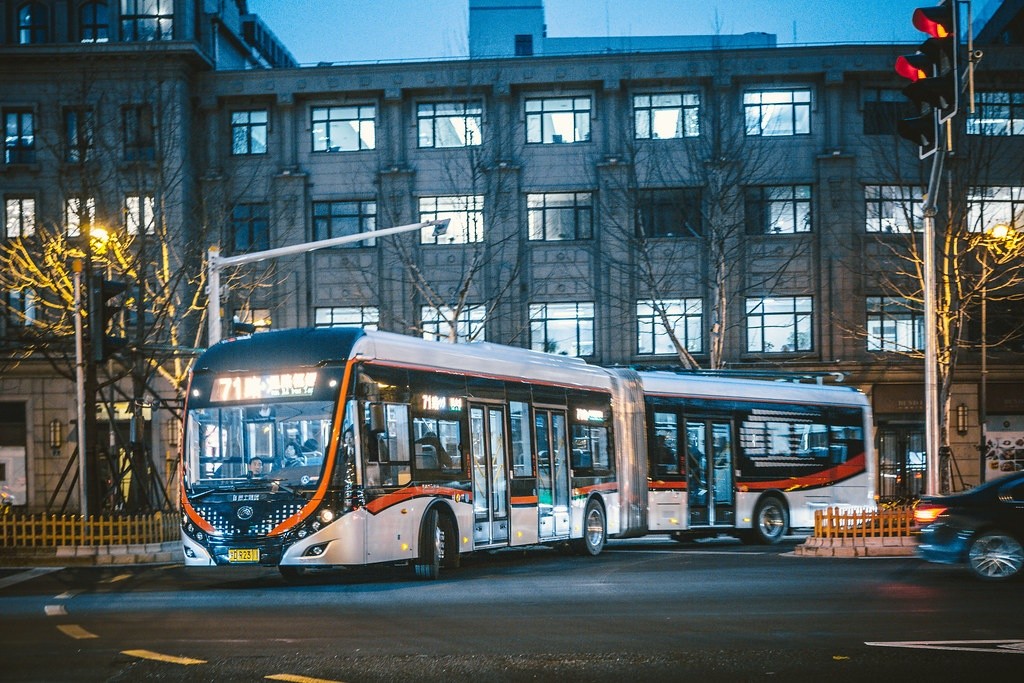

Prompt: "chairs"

[519,449,591,467]
[421,445,439,469]
[301,451,323,483]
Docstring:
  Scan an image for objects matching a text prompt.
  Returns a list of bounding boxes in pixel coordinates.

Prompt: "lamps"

[957,403,969,436]
[50,418,63,455]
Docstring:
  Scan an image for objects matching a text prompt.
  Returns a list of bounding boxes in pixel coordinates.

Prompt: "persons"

[656,434,677,464]
[838,428,850,446]
[424,432,453,469]
[284,442,304,468]
[248,457,263,478]
[688,441,702,503]
[343,428,355,461]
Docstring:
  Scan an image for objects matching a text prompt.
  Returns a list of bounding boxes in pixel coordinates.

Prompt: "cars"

[912,471,1024,587]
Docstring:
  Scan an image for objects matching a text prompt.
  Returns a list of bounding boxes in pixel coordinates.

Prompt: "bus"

[173,327,878,591]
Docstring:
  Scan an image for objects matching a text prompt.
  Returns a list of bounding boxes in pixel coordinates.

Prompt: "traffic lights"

[893,0,969,160]
[90,272,127,363]
